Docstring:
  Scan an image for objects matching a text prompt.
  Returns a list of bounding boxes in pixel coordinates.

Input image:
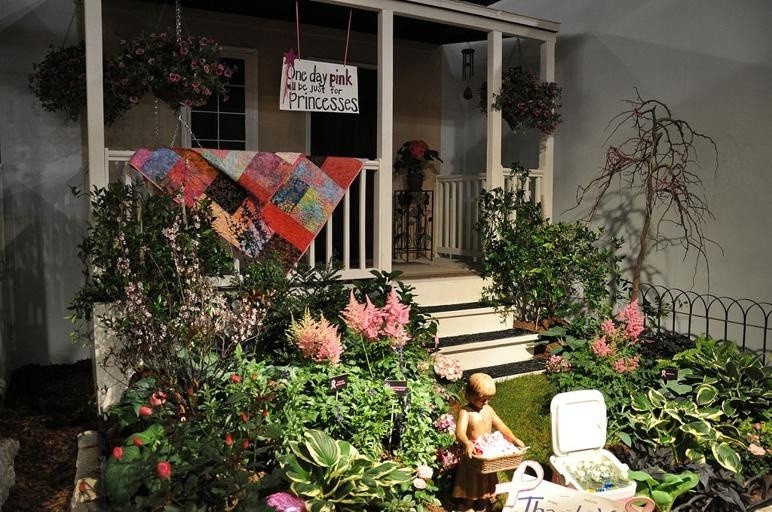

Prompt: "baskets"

[465,446,526,473]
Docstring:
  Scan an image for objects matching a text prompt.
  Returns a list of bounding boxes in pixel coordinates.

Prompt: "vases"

[407,167,424,191]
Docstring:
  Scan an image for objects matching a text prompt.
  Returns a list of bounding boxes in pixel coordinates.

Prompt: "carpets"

[128,147,365,279]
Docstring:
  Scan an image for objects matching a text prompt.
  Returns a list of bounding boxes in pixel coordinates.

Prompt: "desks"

[394,189,434,264]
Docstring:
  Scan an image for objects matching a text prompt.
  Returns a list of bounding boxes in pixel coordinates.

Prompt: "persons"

[454,372,526,508]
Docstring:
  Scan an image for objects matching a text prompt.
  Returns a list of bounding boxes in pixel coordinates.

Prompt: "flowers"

[27,32,237,128]
[79,207,458,512]
[392,140,444,174]
[480,66,566,136]
[543,295,648,383]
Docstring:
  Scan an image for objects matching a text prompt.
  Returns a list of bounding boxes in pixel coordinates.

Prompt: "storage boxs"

[548,388,637,504]
